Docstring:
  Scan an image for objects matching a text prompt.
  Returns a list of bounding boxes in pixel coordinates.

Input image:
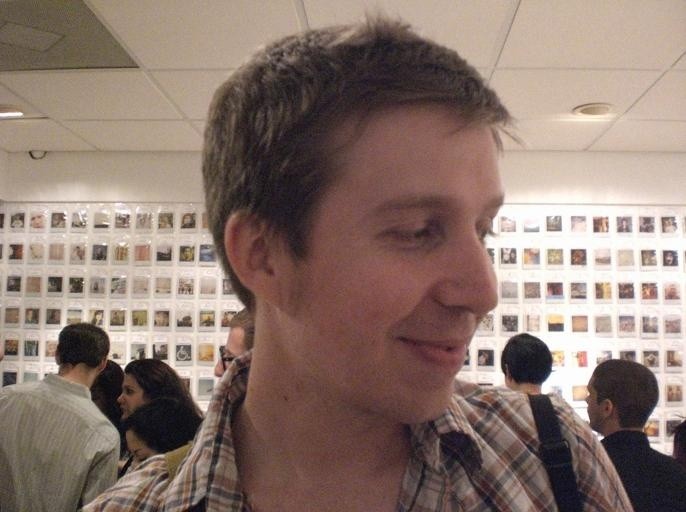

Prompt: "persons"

[665,284,679,300]
[8,212,83,326]
[499,333,553,402]
[501,217,517,234]
[663,218,675,232]
[77,10,636,511]
[89,306,255,480]
[621,221,629,234]
[583,357,684,512]
[0,322,122,511]
[179,213,195,225]
[478,349,493,365]
[669,422,684,463]
[182,246,190,259]
[665,252,677,266]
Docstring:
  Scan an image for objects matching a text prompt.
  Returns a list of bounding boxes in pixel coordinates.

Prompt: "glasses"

[217,345,233,370]
